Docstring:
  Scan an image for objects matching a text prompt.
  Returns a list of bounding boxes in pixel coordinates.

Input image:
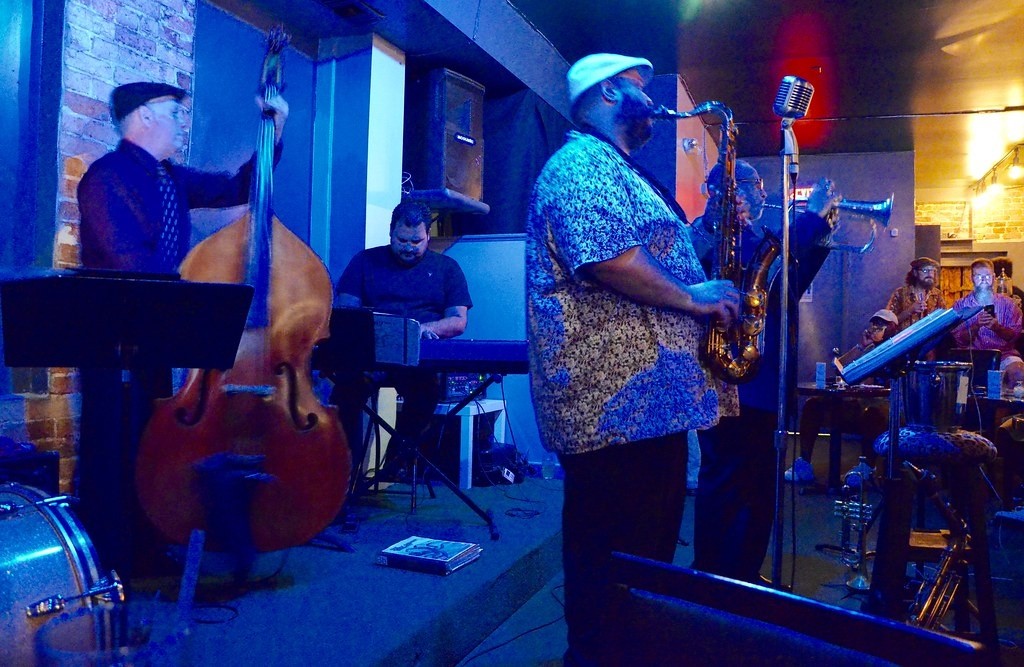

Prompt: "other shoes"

[387,446,412,485]
[844,461,875,490]
[784,456,815,482]
[758,575,793,593]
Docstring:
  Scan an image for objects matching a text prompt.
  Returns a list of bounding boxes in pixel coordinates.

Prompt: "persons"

[524,51,753,667]
[71,81,291,603]
[687,158,847,591]
[783,257,1024,484]
[329,202,474,522]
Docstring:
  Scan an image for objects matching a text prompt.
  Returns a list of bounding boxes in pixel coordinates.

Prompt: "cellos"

[131,22,356,592]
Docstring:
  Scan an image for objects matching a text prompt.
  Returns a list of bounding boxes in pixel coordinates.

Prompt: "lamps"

[1008,148,1024,179]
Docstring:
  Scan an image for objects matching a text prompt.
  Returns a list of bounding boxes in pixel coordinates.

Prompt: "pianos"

[308,339,530,541]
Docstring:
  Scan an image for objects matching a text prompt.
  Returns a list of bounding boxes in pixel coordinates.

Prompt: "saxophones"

[650,100,781,383]
[899,455,988,639]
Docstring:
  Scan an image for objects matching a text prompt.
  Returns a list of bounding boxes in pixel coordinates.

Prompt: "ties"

[158,165,181,266]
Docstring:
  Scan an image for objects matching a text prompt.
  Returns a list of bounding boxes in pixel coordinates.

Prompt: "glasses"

[736,178,764,190]
[869,328,883,332]
[915,267,937,273]
[972,273,993,279]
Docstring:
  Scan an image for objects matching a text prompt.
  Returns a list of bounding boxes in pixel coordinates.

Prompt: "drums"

[0,481,125,665]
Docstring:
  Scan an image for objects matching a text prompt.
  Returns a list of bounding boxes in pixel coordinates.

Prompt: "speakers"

[402,68,485,201]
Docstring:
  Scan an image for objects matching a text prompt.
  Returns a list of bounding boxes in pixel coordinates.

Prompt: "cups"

[987,370,1002,399]
[817,362,827,389]
[36,601,193,667]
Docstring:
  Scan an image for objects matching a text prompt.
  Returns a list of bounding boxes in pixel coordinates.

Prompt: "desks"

[797,380,892,496]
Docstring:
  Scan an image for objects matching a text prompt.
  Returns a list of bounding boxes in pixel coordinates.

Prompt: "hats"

[566,52,654,106]
[910,256,940,267]
[868,309,898,327]
[109,81,187,126]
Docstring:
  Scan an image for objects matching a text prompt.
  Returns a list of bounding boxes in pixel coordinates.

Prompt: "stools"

[433,399,508,493]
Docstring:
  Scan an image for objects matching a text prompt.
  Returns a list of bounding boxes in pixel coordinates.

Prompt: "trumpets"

[834,471,873,591]
[761,178,896,258]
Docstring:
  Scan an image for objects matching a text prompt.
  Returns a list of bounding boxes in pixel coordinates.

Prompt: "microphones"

[772,74,815,119]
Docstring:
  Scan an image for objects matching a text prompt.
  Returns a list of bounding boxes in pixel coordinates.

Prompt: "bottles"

[851,456,873,484]
[1013,380,1024,399]
[541,451,556,479]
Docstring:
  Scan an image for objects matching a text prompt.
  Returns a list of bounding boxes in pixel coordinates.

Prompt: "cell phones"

[984,305,995,318]
